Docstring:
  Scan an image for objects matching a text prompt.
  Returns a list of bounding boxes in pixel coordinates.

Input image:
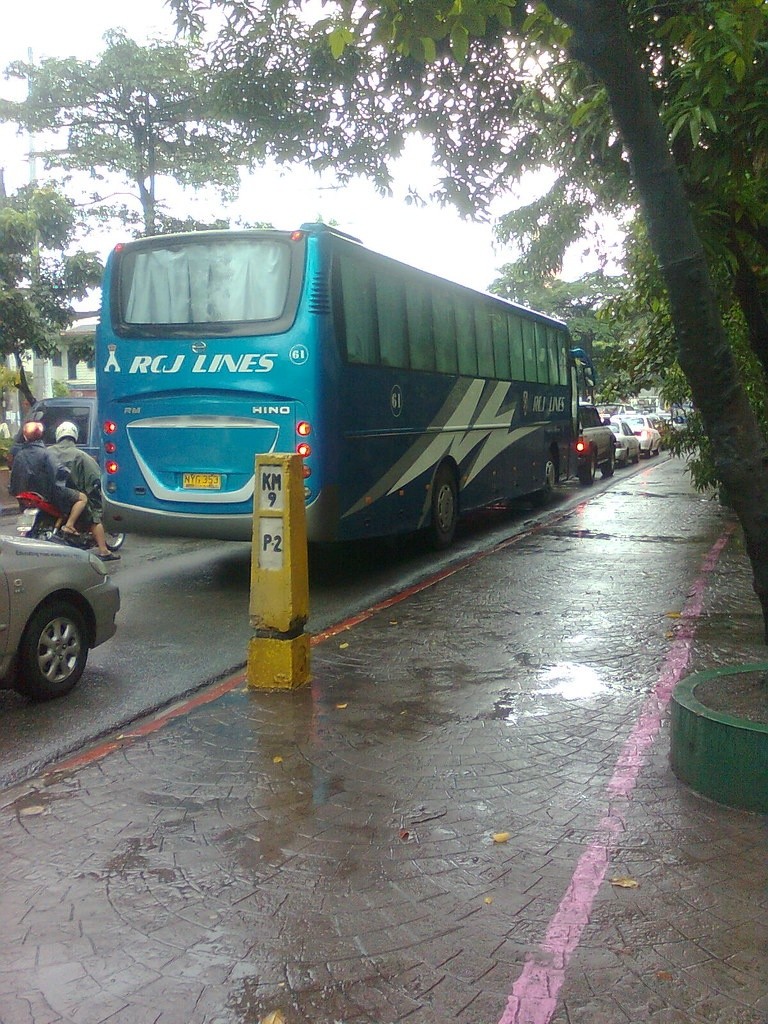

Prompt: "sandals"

[63,526,80,537]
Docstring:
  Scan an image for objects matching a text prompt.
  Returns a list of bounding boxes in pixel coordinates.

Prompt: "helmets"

[55,421,79,442]
[23,421,44,441]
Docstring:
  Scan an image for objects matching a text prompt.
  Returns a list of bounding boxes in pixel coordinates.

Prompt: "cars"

[0,534,121,700]
[579,398,695,481]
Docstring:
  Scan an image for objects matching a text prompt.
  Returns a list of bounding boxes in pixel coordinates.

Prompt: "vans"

[7,397,98,471]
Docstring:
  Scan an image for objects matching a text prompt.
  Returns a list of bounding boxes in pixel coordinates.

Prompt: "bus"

[95,222,594,547]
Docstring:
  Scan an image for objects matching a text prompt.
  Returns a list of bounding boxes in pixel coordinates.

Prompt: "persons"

[10,423,88,538]
[49,423,121,561]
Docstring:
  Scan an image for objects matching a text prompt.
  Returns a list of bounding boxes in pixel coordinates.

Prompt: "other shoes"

[98,551,122,561]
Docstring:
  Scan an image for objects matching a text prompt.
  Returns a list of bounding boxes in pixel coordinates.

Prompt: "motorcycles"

[16,477,124,551]
